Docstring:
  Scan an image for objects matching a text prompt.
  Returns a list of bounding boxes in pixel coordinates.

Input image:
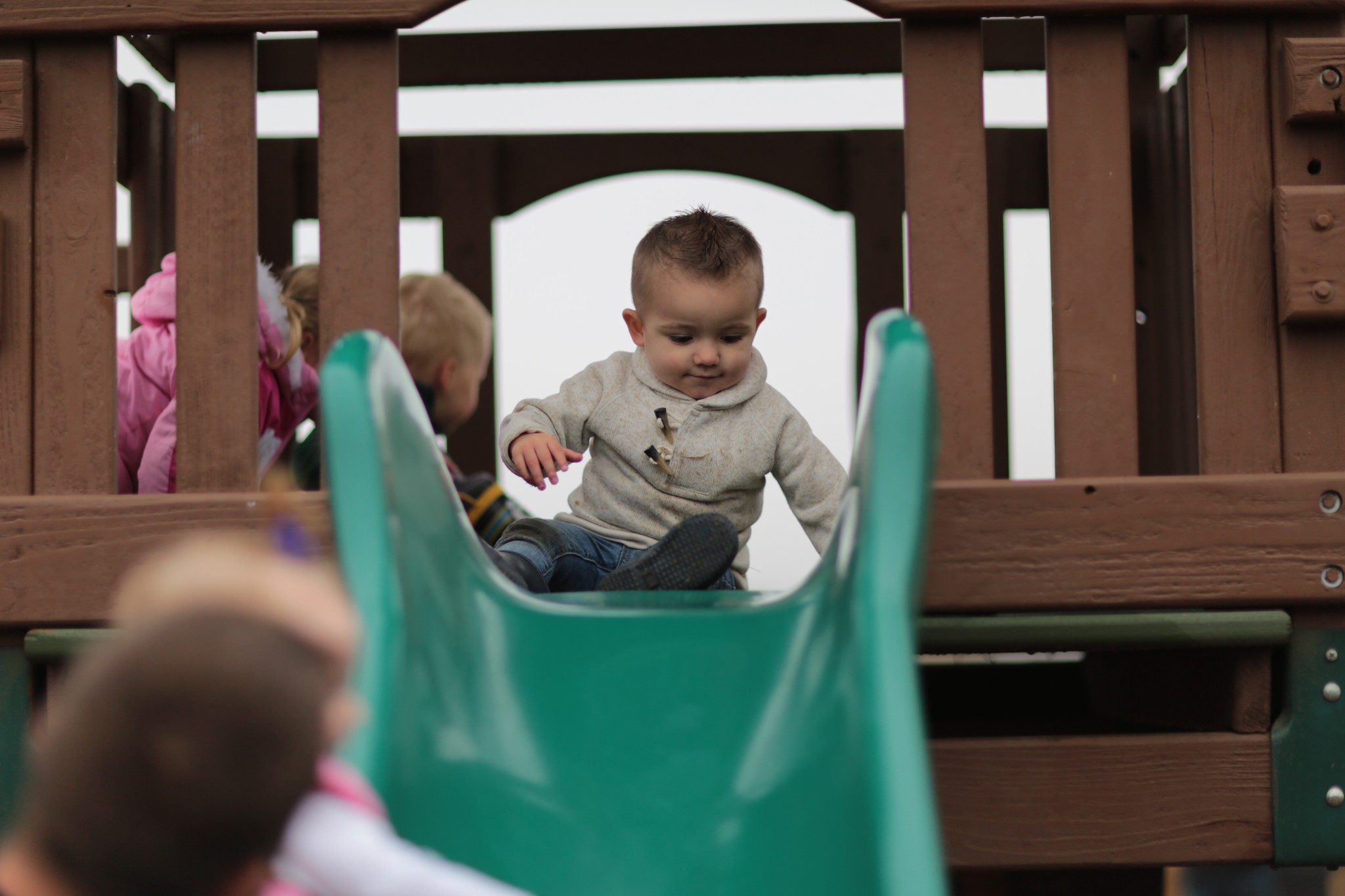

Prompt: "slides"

[319,594,949,895]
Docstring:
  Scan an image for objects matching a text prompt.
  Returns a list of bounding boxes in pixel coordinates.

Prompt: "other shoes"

[479,537,547,594]
[594,512,739,591]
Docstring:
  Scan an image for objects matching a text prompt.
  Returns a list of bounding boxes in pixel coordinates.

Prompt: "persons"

[0,531,538,896]
[117,251,532,549]
[476,205,847,594]
